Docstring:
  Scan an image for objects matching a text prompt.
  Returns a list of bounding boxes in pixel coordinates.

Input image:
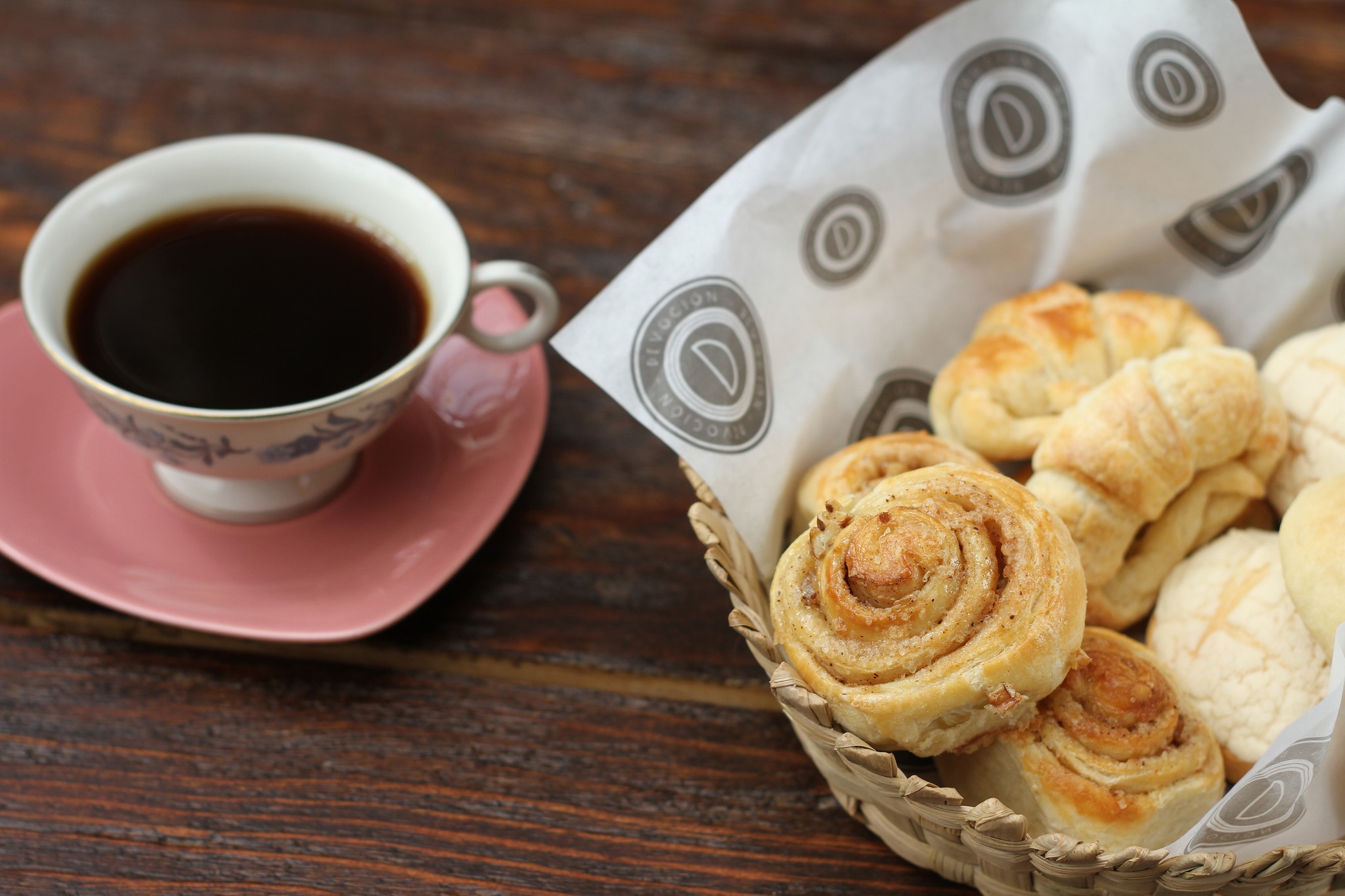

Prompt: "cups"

[17,128,560,522]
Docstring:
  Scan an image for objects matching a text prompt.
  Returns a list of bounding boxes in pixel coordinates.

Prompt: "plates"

[0,261,548,645]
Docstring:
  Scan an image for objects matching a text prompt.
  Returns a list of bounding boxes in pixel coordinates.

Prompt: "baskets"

[674,457,1344,896]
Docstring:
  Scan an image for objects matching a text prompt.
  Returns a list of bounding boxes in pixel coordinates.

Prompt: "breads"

[758,283,1345,855]
